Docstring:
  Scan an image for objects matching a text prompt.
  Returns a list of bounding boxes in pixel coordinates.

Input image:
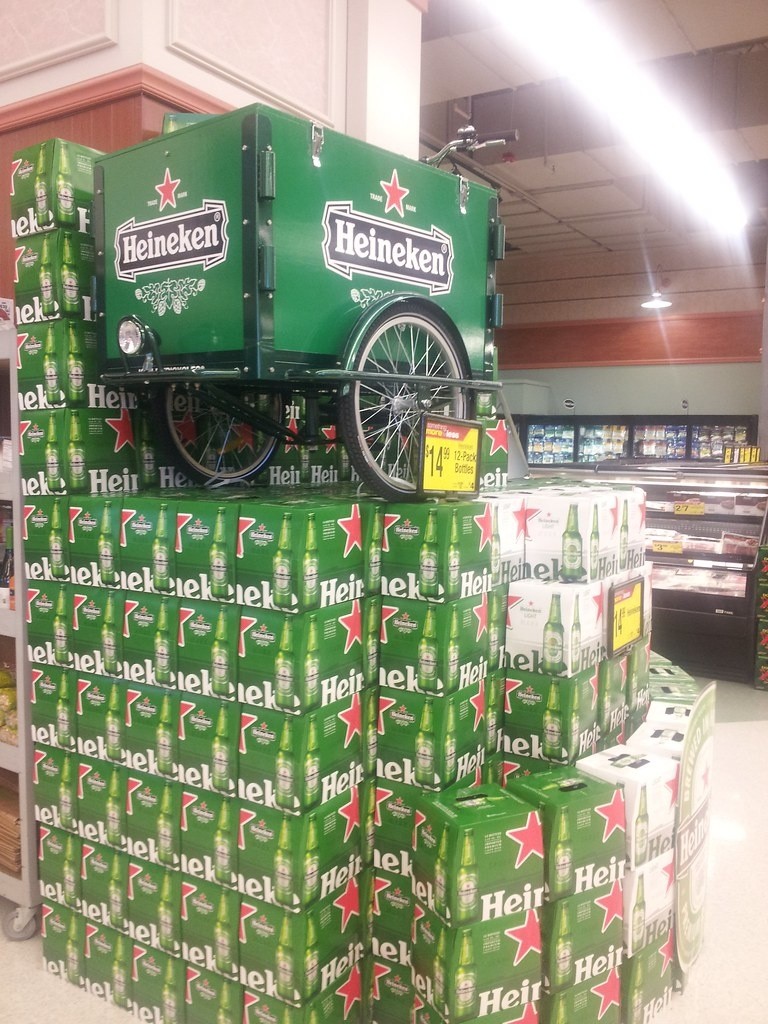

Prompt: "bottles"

[35,139,648,1022]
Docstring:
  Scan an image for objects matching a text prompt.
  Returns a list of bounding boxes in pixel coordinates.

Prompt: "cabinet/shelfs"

[0,296,39,940]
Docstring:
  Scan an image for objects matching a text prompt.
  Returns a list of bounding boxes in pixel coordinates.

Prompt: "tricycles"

[88,101,520,506]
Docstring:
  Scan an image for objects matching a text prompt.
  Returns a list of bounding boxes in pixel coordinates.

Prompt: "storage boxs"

[10,137,702,1024]
